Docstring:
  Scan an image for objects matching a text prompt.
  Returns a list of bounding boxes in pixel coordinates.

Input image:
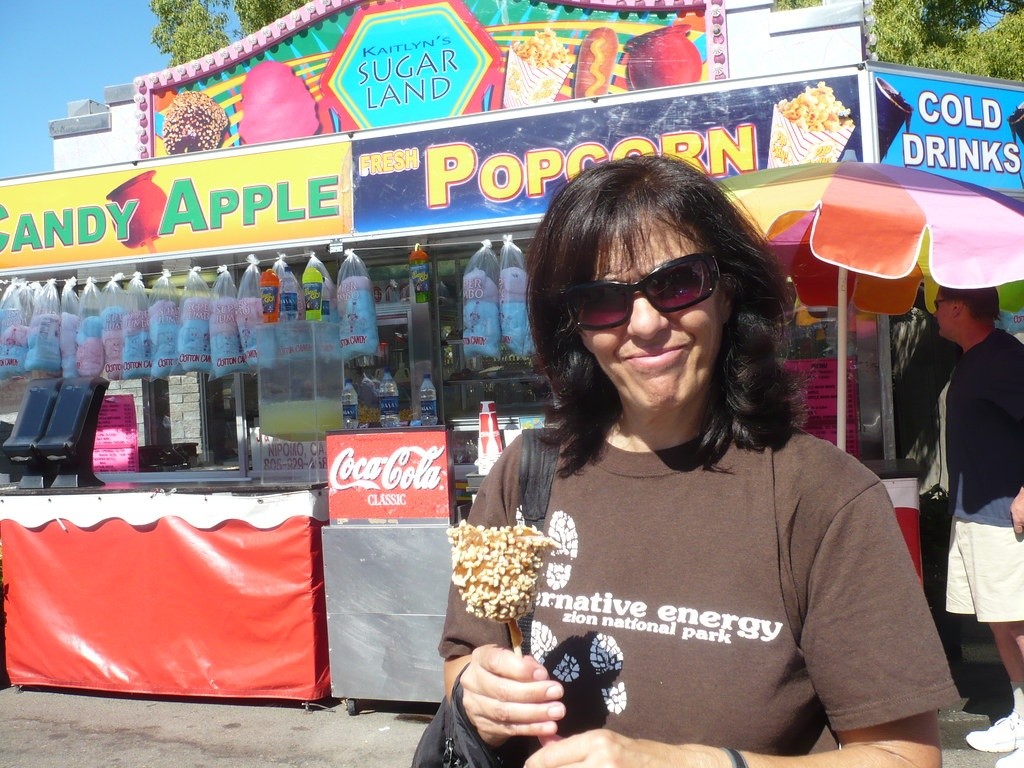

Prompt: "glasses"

[934,299,968,311]
[561,254,721,330]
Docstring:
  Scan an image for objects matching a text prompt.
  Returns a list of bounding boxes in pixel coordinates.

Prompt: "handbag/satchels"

[411,663,505,768]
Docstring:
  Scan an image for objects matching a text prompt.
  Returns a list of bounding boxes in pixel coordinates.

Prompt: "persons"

[931,287,1024,768]
[437,154,962,768]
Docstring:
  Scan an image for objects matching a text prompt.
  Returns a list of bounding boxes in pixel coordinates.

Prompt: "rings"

[1014,525,1023,534]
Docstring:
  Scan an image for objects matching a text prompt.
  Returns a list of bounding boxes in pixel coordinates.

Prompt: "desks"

[0,482,325,713]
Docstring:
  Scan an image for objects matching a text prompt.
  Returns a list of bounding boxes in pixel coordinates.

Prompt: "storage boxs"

[786,355,863,461]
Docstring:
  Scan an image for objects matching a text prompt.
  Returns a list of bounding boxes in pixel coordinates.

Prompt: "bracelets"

[721,747,749,768]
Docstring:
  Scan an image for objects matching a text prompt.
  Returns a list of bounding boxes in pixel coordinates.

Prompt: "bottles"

[260,271,281,323]
[409,243,431,303]
[341,379,358,430]
[302,267,323,322]
[420,372,438,426]
[378,367,401,428]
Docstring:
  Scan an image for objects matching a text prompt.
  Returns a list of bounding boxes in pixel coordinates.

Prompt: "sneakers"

[995,747,1024,768]
[966,712,1024,753]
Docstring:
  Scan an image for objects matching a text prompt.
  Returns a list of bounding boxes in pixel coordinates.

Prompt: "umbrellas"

[711,163,1024,455]
